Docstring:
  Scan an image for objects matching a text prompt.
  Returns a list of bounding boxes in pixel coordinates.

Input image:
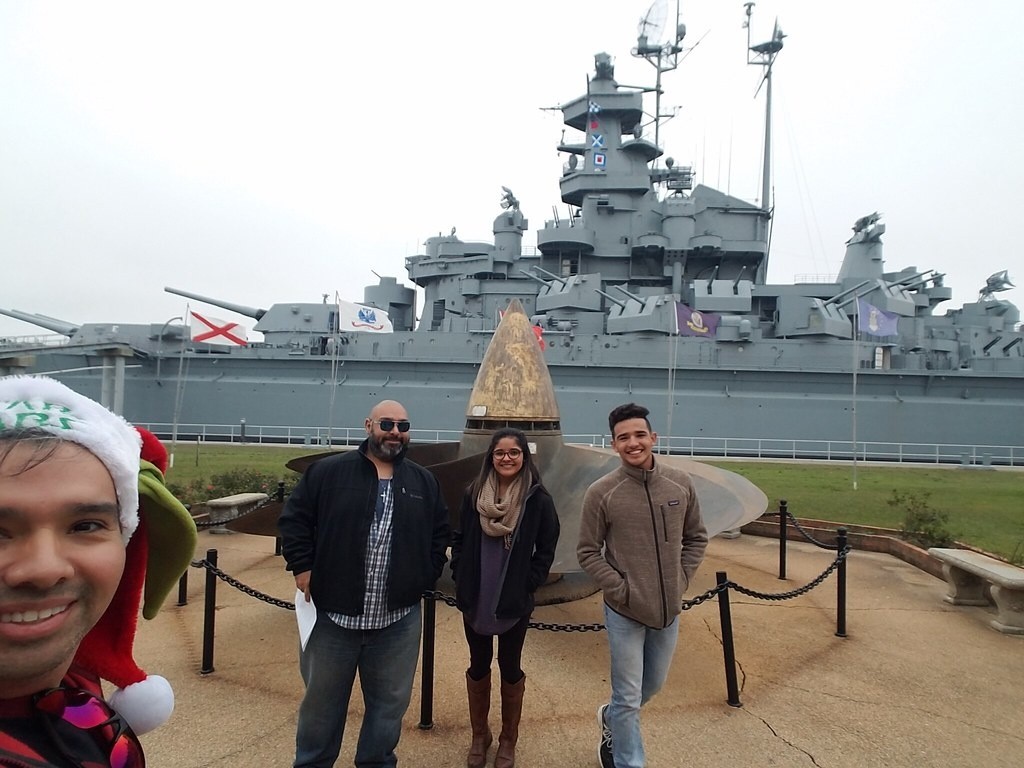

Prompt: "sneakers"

[597,703,616,768]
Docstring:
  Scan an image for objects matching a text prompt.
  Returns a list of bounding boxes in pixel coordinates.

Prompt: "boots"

[495,673,527,768]
[465,668,493,768]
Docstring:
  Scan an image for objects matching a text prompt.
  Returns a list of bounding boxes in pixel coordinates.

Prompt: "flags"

[189,310,248,348]
[338,298,394,334]
[674,300,721,339]
[500,308,545,351]
[855,296,900,336]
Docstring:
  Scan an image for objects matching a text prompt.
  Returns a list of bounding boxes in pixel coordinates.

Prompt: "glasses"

[27,685,146,768]
[492,449,523,461]
[372,420,410,432]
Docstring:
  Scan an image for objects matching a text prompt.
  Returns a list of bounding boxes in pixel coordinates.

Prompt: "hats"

[0,374,175,738]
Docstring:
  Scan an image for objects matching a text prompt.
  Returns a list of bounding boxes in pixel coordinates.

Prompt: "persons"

[576,400,711,767]
[0,373,198,767]
[275,397,464,768]
[449,425,562,767]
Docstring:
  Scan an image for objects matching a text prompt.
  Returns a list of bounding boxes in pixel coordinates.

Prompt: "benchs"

[928,547,1024,634]
[206,493,270,534]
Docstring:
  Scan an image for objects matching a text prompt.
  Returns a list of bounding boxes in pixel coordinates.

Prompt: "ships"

[0,3,1024,462]
